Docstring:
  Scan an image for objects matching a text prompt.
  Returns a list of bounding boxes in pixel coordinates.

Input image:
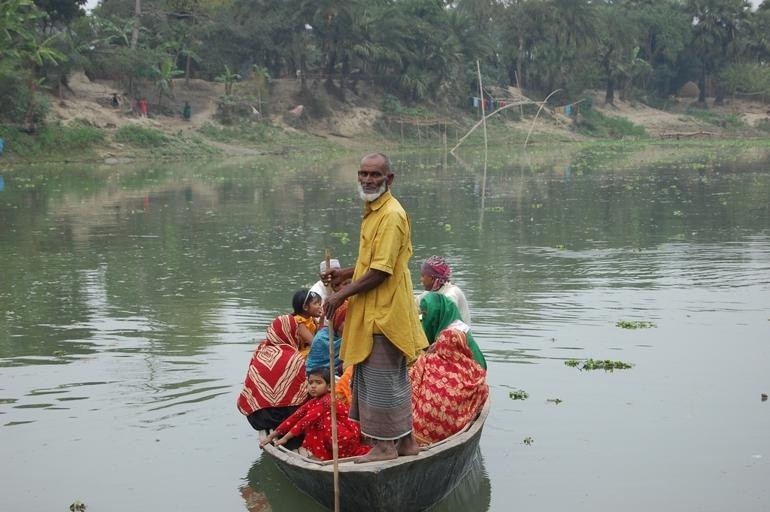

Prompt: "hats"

[320,259,341,272]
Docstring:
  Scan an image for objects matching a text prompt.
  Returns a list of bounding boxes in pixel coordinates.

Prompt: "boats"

[259,314,491,512]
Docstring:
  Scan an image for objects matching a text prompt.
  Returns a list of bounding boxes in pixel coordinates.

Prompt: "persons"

[184,102,191,120]
[419,292,487,371]
[420,255,471,328]
[320,153,430,464]
[407,329,489,447]
[237,258,373,461]
[111,94,147,118]
